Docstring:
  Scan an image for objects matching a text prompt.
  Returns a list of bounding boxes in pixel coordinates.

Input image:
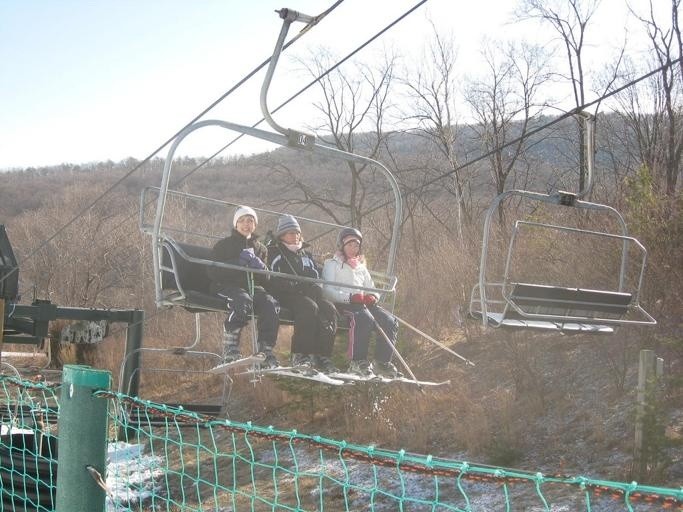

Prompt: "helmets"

[336,227,363,255]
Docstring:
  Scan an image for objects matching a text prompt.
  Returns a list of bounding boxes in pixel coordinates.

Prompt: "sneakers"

[257,344,280,371]
[221,324,244,365]
[370,358,402,379]
[315,353,340,379]
[289,351,317,378]
[345,358,374,382]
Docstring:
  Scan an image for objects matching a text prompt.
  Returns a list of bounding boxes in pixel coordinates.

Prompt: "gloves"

[364,294,377,305]
[301,265,319,278]
[239,250,267,270]
[351,294,365,304]
[290,279,304,289]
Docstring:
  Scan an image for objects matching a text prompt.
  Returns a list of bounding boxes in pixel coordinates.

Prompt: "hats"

[275,213,302,239]
[233,204,258,230]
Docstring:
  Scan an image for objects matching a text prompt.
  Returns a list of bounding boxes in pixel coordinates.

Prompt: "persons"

[317,225,401,379]
[264,212,340,380]
[202,203,282,369]
